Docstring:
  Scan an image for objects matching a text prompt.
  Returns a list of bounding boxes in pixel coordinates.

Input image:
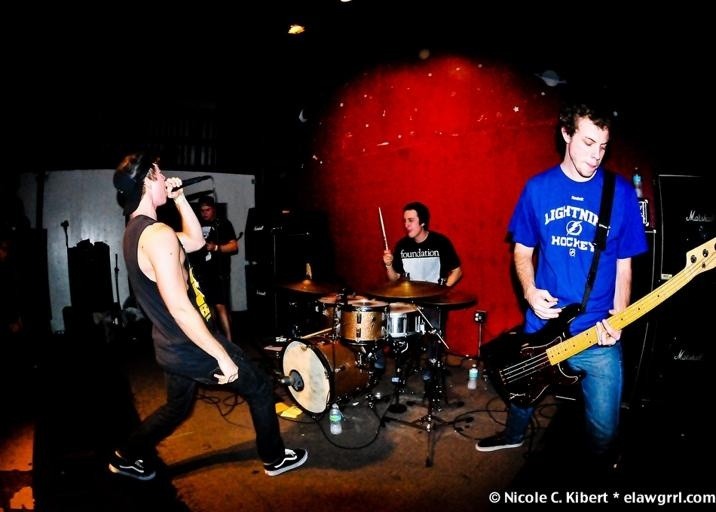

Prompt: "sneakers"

[263,449,308,476]
[109,451,156,480]
[475,433,524,452]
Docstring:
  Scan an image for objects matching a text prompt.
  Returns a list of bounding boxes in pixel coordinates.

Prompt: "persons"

[191,193,238,346]
[0,219,42,424]
[106,152,309,481]
[383,202,463,407]
[473,100,648,470]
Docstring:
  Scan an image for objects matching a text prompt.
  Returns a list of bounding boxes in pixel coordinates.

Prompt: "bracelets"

[214,244,218,252]
[522,282,536,302]
[385,263,392,268]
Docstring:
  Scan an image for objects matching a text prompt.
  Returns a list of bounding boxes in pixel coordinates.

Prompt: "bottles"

[329,403,343,436]
[632,168,643,198]
[467,364,478,390]
[274,351,281,372]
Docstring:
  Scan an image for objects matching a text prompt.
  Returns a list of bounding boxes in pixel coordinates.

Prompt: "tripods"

[334,361,391,427]
[383,330,474,467]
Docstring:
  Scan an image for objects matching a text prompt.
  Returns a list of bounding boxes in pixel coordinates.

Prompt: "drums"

[282,338,374,417]
[341,300,390,345]
[387,304,424,338]
[314,295,369,336]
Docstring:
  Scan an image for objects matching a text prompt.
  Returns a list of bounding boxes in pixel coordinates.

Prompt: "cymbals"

[425,292,477,305]
[483,237,716,407]
[365,282,449,298]
[281,279,346,293]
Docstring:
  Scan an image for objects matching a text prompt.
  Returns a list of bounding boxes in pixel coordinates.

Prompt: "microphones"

[166,175,210,193]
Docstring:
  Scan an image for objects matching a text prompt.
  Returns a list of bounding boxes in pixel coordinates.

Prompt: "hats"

[114,153,153,218]
[198,196,214,206]
